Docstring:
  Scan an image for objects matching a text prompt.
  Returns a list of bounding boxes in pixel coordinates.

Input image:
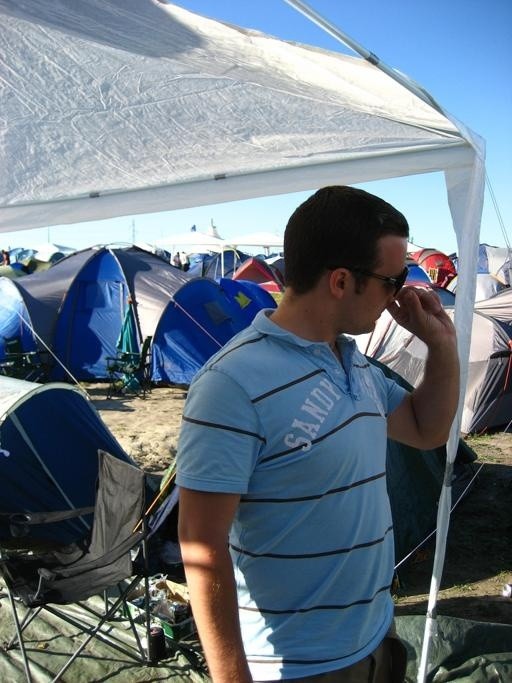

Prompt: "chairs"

[0,449,153,682]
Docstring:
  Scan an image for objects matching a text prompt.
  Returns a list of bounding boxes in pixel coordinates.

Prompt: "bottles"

[149,626,165,659]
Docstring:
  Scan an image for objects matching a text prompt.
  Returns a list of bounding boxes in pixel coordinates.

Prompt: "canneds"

[146,627,165,660]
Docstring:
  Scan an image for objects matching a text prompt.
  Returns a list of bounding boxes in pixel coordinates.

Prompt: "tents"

[1,218,510,437]
[0,370,153,588]
[127,355,479,591]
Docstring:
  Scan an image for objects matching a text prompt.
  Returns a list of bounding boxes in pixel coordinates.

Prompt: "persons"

[175,184,460,682]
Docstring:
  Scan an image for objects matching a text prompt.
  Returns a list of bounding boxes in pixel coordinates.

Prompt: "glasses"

[355,267,408,297]
